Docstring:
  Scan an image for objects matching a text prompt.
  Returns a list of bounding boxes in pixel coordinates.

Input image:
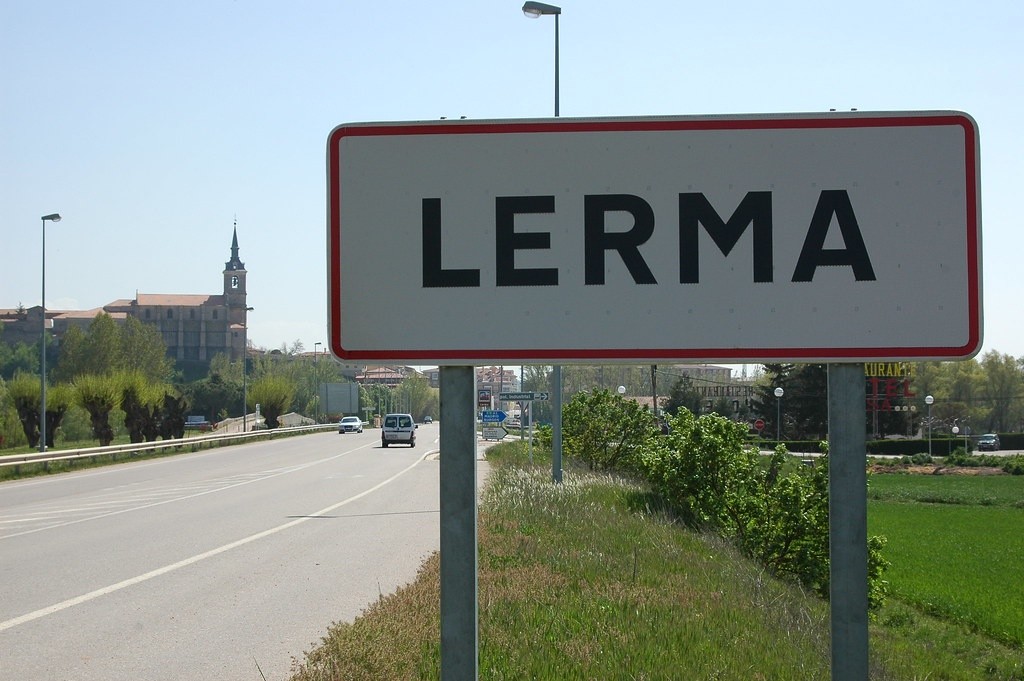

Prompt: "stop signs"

[753,420,765,430]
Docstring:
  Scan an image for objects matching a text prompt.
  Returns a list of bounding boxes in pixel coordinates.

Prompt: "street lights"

[618,387,626,396]
[314,342,321,424]
[952,428,959,439]
[525,0,563,480]
[41,213,62,448]
[926,396,934,456]
[243,306,254,431]
[774,387,784,449]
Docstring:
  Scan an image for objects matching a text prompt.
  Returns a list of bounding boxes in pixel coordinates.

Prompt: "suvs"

[979,434,1001,450]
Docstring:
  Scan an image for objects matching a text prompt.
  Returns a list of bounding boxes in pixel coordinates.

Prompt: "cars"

[424,416,433,424]
[338,416,363,434]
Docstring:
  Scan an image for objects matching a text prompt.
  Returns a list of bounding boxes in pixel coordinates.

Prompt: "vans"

[380,414,418,448]
